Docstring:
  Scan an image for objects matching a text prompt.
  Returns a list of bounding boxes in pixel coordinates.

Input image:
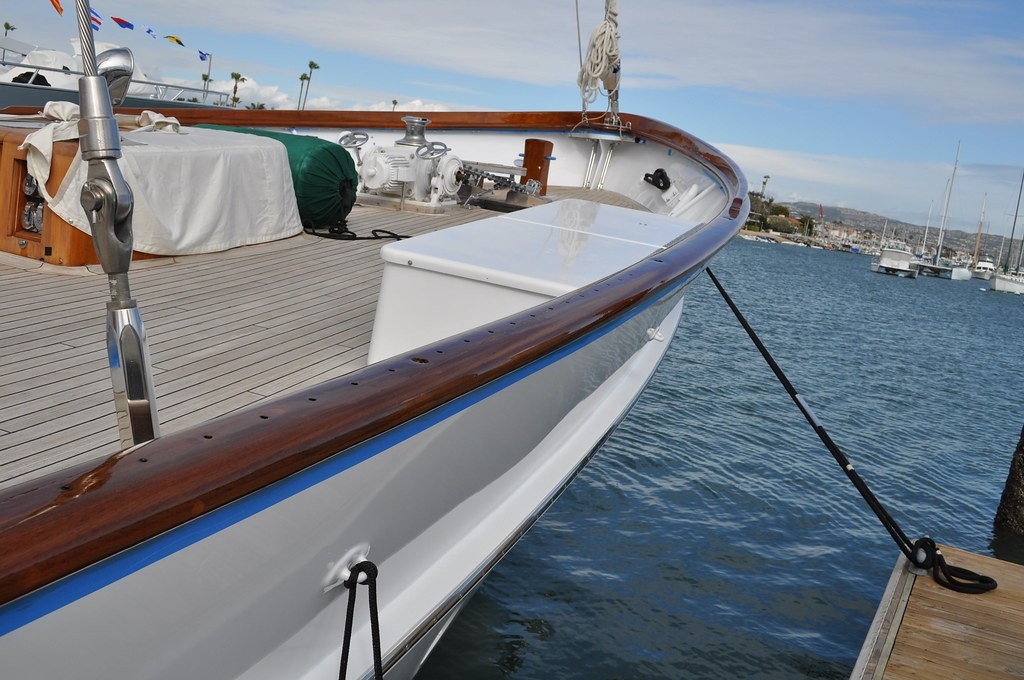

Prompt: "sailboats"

[918,140,972,280]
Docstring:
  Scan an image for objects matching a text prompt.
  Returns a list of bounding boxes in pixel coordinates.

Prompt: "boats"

[1,82,751,679]
[988,272,1024,295]
[974,254,995,280]
[870,247,918,279]
